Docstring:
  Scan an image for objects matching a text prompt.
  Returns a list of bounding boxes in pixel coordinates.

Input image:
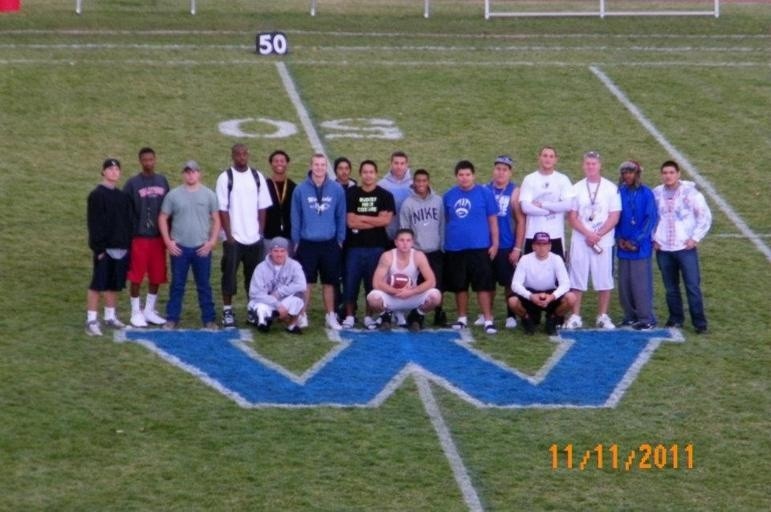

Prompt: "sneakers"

[257,321,269,334]
[142,310,167,325]
[617,316,707,334]
[203,321,220,331]
[83,319,103,338]
[221,308,235,329]
[286,324,304,335]
[595,313,616,331]
[161,320,177,330]
[324,312,343,331]
[298,313,309,327]
[130,311,148,328]
[246,311,257,325]
[335,311,564,337]
[562,314,583,330]
[103,316,125,329]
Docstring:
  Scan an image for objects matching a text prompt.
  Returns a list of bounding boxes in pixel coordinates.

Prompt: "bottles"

[594,244,604,255]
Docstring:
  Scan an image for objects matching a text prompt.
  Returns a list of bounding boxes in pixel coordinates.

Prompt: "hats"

[181,160,201,174]
[494,154,514,169]
[617,159,642,188]
[99,158,121,176]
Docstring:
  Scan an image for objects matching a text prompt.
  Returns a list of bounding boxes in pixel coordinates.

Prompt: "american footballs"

[385,274,410,290]
